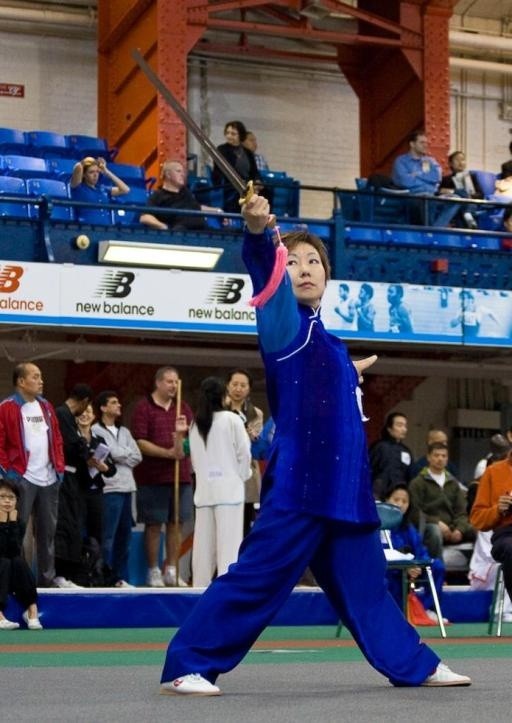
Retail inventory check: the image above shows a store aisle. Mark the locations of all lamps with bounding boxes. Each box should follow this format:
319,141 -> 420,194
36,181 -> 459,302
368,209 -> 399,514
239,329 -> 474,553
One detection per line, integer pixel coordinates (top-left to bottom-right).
97,237 -> 225,271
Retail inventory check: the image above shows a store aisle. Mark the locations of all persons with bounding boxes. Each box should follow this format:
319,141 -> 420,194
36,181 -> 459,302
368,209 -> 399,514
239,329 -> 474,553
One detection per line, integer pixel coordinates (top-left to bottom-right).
212,121 -> 262,213
392,131 -> 462,227
69,156 -> 130,226
439,151 -> 485,229
242,132 -> 268,171
160,194 -> 472,697
139,161 -> 232,229
493,162 -> 512,231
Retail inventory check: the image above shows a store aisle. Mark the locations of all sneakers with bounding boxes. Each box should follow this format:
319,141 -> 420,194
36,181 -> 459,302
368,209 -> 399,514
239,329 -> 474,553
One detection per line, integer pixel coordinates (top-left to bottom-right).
162,565 -> 188,586
114,579 -> 130,588
159,672 -> 224,699
0,616 -> 20,630
145,569 -> 166,588
425,609 -> 449,624
22,609 -> 42,630
38,573 -> 83,588
419,662 -> 472,688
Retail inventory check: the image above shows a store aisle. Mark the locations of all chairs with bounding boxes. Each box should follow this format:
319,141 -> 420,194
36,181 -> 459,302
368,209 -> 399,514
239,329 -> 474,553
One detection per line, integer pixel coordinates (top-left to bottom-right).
338,501 -> 450,639
308,169 -> 510,250
1,129 -> 151,225
188,163 -> 303,240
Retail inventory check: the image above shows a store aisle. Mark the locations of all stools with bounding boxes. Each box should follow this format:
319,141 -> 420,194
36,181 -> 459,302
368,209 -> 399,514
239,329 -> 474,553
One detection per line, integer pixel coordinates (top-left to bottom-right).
486,563 -> 511,639
